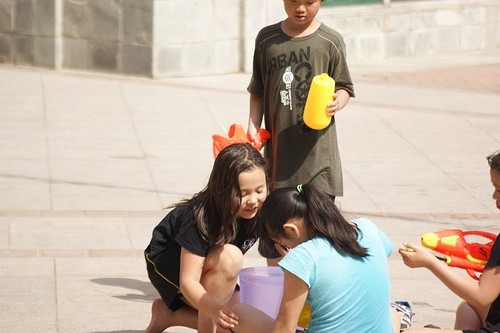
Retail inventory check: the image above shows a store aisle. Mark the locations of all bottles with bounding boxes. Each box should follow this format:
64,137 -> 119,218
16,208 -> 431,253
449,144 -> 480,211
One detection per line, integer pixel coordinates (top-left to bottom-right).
303,73 -> 337,131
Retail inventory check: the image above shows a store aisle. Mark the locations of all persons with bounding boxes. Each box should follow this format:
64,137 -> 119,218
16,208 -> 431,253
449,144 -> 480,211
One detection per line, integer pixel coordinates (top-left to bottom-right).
230,185 -> 394,333
245,1 -> 355,267
397,151 -> 500,333
145,144 -> 268,333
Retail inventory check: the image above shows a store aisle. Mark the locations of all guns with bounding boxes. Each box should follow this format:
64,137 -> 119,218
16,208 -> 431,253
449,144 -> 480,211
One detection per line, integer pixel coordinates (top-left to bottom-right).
212,123 -> 271,161
400,228 -> 497,280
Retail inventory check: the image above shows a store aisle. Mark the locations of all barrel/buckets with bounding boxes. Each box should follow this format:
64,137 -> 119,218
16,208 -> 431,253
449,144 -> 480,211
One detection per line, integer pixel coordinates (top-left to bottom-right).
238,266 -> 285,321
